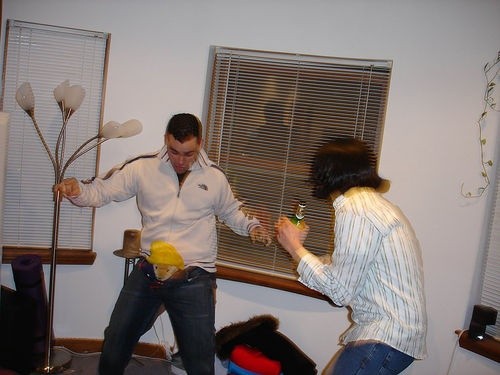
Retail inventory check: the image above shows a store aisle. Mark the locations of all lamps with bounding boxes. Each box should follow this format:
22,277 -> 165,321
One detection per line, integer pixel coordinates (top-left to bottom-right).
16,80 -> 142,375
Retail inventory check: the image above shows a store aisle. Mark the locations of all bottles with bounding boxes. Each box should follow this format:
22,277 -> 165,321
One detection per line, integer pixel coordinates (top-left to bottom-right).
290,200 -> 307,230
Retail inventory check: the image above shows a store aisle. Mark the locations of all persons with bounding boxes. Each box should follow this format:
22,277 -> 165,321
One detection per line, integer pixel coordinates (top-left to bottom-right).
274,135 -> 428,375
52,113 -> 272,375
